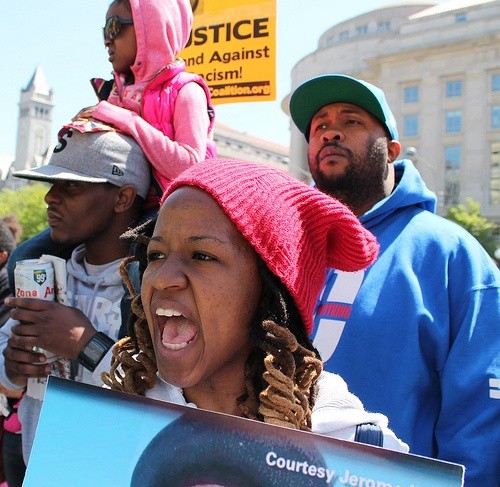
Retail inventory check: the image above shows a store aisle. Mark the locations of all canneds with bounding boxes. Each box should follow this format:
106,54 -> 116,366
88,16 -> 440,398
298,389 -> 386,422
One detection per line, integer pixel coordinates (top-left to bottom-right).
14,258 -> 61,365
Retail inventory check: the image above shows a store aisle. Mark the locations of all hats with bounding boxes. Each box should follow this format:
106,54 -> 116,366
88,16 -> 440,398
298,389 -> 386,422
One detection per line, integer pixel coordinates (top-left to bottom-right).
160,158 -> 380,336
13,131 -> 149,199
289,73 -> 399,143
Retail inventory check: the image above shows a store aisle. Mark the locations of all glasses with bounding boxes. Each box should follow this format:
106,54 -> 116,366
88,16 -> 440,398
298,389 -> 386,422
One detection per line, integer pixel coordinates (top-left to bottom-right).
102,11 -> 133,41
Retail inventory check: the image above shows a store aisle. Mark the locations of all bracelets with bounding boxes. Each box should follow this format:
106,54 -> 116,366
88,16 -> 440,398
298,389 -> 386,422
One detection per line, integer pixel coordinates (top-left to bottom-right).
76,331 -> 116,373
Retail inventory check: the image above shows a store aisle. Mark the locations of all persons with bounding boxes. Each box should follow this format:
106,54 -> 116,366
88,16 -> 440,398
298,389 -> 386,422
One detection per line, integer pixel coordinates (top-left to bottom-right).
290,74 -> 499,487
100,160 -> 409,452
71,0 -> 215,190
0,129 -> 152,487
